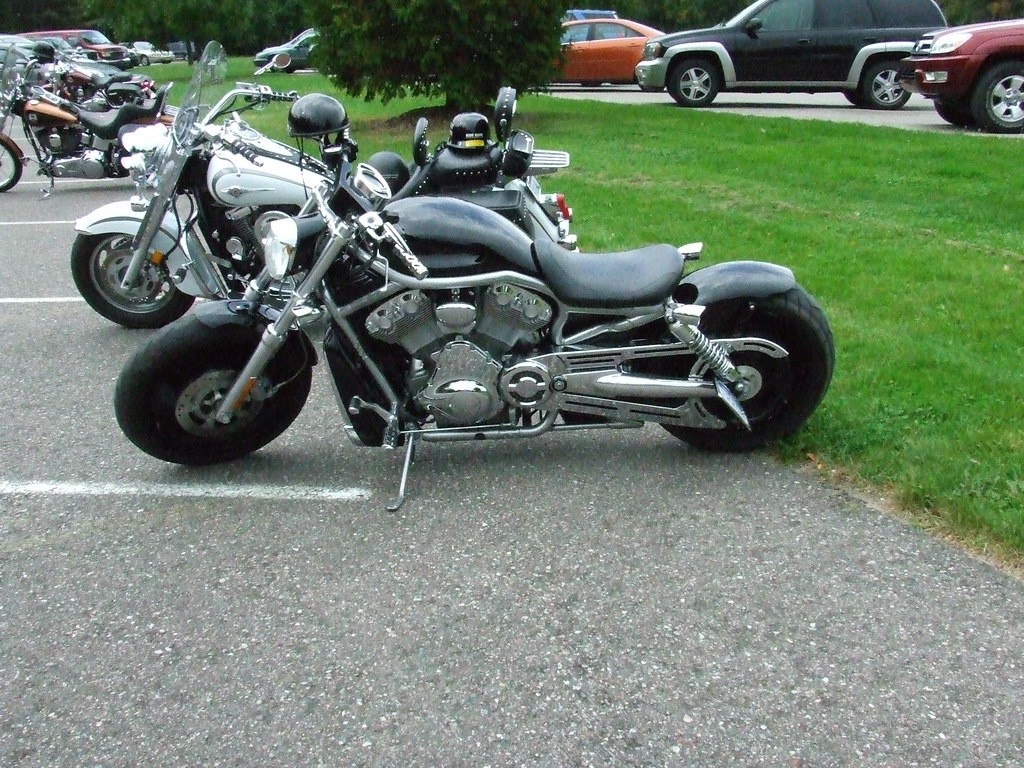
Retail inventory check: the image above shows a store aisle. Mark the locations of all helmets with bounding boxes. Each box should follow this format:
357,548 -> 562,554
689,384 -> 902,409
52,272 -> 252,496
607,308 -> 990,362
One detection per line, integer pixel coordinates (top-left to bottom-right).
288,93 -> 350,136
32,40 -> 55,59
445,112 -> 499,149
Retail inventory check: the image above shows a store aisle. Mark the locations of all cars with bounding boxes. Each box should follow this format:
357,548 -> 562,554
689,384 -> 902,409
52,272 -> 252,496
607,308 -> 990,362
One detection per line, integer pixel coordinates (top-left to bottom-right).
551,19 -> 667,85
0,35 -> 100,79
253,27 -> 319,74
118,42 -> 175,66
166,42 -> 195,61
898,19 -> 1024,135
561,10 -> 629,87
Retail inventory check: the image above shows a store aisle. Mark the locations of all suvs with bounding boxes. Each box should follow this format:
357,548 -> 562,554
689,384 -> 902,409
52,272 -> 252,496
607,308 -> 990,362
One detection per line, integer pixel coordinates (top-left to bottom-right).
634,0 -> 949,110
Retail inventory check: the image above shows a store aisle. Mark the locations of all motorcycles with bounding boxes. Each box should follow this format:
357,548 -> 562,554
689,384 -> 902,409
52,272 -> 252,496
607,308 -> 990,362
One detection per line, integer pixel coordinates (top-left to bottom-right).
114,127 -> 836,511
71,40 -> 580,329
0,40 -> 188,198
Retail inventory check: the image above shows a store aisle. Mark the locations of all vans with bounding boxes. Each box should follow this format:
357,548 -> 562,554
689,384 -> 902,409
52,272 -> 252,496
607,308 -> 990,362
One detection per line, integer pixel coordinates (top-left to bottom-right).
15,30 -> 132,71
